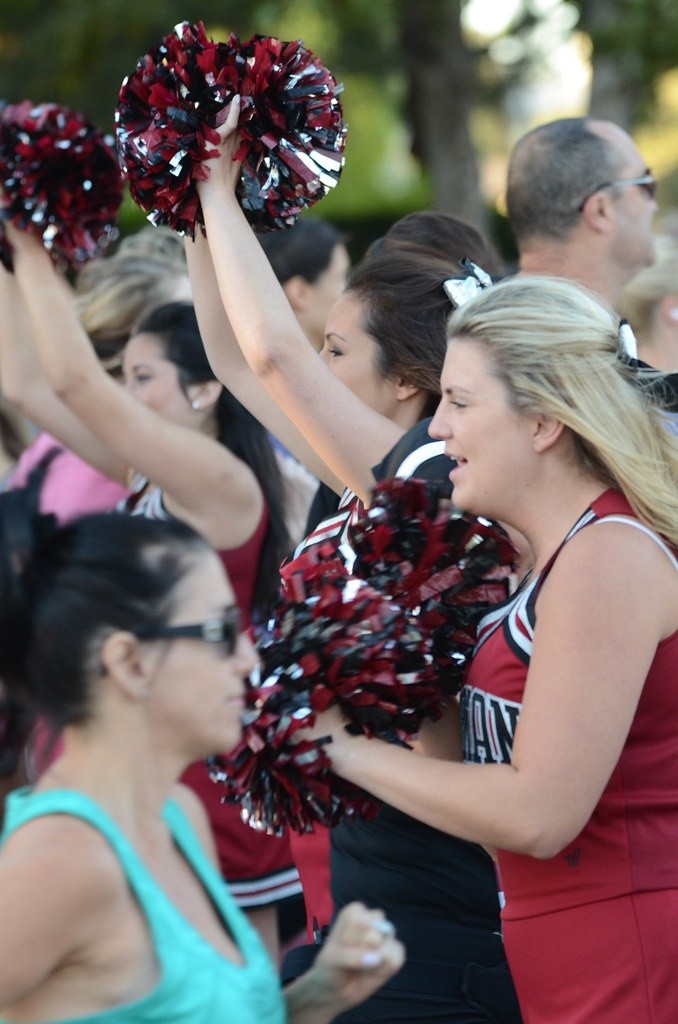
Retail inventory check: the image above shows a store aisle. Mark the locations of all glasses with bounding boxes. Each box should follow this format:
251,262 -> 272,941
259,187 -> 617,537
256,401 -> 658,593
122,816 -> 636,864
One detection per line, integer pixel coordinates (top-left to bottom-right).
579,167 -> 656,212
99,605 -> 243,676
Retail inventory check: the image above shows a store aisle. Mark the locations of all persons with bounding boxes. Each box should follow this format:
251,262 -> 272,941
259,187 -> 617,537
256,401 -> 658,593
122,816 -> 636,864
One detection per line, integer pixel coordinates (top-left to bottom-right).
0,94 -> 678,1024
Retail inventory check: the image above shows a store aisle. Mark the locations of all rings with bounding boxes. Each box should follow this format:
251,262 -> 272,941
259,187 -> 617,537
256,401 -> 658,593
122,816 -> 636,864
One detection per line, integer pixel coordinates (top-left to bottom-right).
371,918 -> 395,939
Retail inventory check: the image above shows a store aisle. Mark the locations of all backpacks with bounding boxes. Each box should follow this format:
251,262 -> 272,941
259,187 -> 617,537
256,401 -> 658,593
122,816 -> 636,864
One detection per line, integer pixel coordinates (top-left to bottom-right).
0,449 -> 74,689
